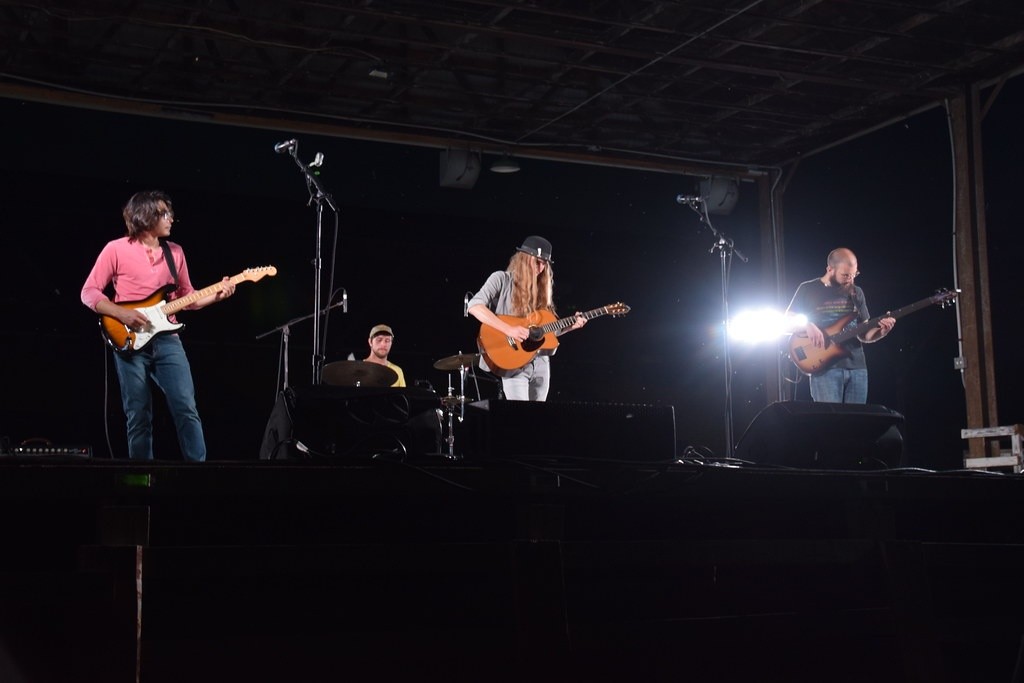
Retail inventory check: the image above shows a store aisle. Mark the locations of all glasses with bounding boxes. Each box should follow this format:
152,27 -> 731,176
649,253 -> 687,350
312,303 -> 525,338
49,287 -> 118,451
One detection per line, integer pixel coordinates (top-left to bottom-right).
159,210 -> 175,220
840,270 -> 860,280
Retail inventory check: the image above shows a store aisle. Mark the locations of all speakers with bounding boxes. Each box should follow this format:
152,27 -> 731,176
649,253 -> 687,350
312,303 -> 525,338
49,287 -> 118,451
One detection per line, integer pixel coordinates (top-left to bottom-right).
486,401 -> 676,465
736,400 -> 905,467
259,386 -> 440,463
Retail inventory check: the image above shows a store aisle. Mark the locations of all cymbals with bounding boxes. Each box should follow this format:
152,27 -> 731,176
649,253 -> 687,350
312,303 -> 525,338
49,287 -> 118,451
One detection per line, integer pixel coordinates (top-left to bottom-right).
321,360 -> 399,386
433,354 -> 479,370
440,396 -> 474,405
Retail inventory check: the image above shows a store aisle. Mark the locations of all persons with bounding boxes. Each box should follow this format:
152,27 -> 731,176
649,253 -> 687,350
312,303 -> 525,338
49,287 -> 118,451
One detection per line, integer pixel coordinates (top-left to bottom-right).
359,324 -> 409,386
467,236 -> 587,402
80,192 -> 236,462
784,248 -> 897,403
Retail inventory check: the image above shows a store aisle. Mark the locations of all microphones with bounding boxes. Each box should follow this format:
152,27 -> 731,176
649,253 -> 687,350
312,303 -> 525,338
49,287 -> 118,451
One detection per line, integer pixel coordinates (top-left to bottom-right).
677,194 -> 702,204
464,294 -> 469,317
274,139 -> 297,153
343,290 -> 347,313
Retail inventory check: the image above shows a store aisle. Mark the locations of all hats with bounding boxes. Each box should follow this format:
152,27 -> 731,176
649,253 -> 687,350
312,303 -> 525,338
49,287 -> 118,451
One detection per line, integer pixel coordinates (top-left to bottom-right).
515,236 -> 554,265
369,324 -> 394,339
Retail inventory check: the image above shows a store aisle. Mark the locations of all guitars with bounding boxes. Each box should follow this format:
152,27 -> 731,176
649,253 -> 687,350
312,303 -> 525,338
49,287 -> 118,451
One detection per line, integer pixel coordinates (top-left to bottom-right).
98,264 -> 278,359
477,302 -> 631,378
788,286 -> 961,376
